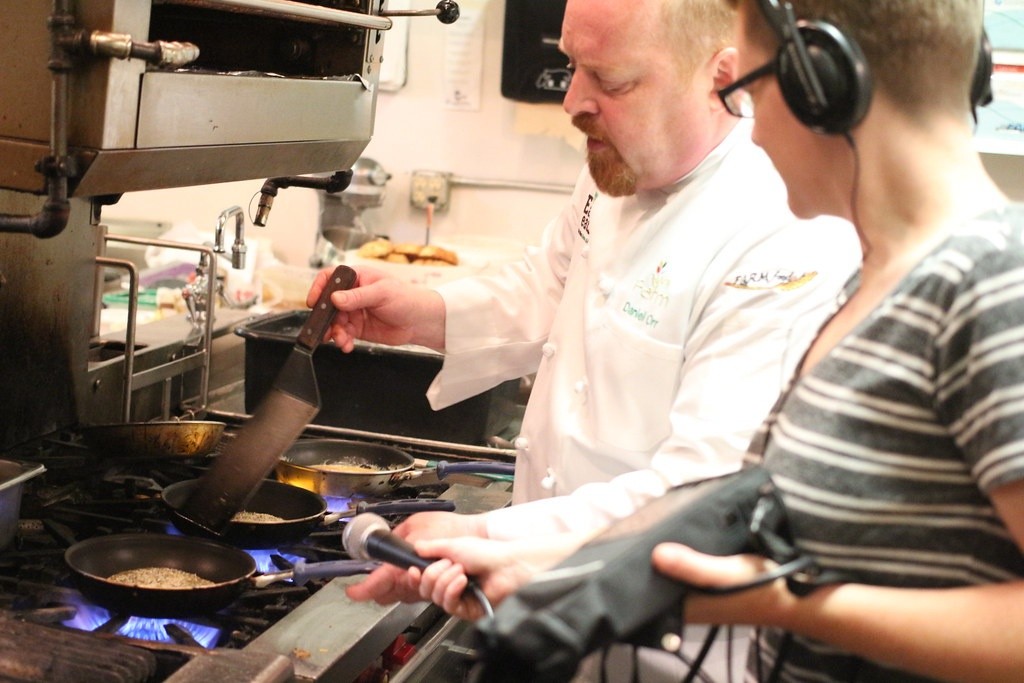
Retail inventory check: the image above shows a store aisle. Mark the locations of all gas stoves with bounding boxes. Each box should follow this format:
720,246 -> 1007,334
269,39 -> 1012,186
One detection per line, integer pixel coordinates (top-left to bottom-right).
0,403 -> 515,683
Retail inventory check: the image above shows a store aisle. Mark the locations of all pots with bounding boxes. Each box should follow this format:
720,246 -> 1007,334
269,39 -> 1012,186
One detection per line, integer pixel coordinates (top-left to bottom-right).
64,532 -> 382,619
84,421 -> 226,465
161,478 -> 455,549
278,438 -> 516,499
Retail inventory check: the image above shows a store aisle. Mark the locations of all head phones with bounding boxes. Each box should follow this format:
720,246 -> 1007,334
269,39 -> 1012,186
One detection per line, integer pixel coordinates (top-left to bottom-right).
760,0 -> 993,135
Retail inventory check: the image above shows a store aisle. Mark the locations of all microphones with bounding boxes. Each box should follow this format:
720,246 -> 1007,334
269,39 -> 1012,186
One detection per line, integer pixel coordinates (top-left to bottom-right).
342,513 -> 480,599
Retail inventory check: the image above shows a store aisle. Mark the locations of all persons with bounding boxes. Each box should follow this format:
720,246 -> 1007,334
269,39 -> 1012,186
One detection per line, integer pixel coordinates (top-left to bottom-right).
307,2 -> 865,683
408,1 -> 1022,683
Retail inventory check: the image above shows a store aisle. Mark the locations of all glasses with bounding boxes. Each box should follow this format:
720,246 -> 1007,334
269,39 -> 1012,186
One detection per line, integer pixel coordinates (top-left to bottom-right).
717,61 -> 775,118
